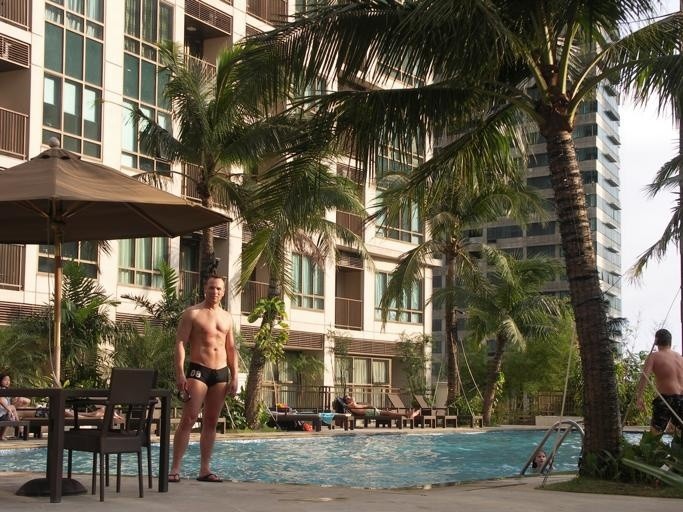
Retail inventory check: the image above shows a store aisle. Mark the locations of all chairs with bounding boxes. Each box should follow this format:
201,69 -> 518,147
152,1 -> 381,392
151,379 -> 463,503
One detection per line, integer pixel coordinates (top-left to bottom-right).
63,365 -> 160,504
121,383 -> 486,436
19,415 -> 125,436
0,417 -> 34,442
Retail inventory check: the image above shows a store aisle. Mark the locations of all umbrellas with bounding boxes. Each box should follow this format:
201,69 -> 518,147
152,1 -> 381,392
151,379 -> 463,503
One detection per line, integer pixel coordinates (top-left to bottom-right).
0,137 -> 236,387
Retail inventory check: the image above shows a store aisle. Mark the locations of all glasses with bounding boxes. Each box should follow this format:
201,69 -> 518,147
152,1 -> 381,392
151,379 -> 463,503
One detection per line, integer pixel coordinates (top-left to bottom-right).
177,389 -> 191,402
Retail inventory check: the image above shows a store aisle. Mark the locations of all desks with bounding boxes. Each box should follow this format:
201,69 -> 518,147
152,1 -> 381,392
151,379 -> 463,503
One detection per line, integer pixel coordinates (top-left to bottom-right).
0,388 -> 174,503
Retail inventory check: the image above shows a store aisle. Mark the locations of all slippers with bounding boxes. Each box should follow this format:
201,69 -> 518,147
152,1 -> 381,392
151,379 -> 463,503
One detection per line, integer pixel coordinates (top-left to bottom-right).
167,473 -> 180,482
197,473 -> 223,482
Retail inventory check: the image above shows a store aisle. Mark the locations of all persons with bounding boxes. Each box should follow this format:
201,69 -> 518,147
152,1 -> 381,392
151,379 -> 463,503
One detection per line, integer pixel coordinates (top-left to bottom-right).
167,273 -> 238,482
10,396 -> 122,420
636,329 -> 681,443
532,449 -> 555,470
344,394 -> 420,419
0,375 -> 23,440
